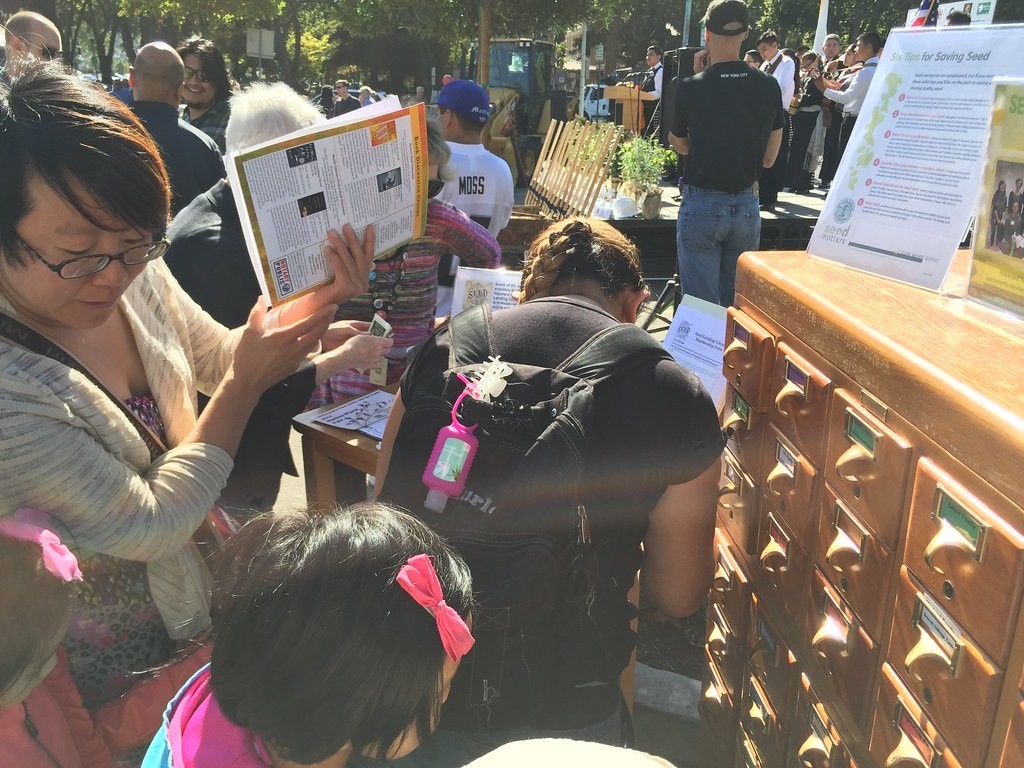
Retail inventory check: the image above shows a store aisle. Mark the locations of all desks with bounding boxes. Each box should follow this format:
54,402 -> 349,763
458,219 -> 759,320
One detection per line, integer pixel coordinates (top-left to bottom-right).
293,382 -> 402,516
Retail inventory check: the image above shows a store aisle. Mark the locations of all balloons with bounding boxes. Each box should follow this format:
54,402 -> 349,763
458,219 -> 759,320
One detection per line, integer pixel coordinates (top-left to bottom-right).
442,74 -> 453,85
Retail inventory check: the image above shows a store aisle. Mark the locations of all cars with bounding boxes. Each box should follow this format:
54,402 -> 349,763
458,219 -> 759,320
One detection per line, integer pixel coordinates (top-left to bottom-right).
583,83 -> 610,124
609,67 -> 653,91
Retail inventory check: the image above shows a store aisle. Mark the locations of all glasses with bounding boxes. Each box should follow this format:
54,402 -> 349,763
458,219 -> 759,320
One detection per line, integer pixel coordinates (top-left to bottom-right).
337,86 -> 343,89
183,66 -> 213,82
800,59 -> 814,72
428,175 -> 444,199
746,59 -> 756,63
18,39 -> 67,61
13,231 -> 170,282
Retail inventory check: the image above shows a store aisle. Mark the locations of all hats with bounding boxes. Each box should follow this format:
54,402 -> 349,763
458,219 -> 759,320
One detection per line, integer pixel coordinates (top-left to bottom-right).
441,75 -> 453,84
437,79 -> 490,123
699,0 -> 749,36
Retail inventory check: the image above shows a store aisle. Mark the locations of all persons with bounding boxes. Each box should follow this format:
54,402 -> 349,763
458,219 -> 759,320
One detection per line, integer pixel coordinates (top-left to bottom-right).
343,217 -> 726,768
669,0 -> 783,306
991,177 -> 1024,246
0,507 -> 216,768
408,87 -> 426,106
163,81 -> 390,516
0,62 -> 375,768
431,80 -> 513,317
0,11 -> 231,224
294,119 -> 502,509
134,500 -> 473,768
315,79 -> 376,119
640,12 -> 971,209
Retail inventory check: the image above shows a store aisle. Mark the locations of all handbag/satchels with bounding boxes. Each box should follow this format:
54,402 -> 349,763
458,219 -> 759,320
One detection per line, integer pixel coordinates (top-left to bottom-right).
51,503 -> 237,765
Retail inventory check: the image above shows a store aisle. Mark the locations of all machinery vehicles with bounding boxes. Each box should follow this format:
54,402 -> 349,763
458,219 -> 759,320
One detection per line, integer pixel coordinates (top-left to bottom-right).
467,35 -> 580,190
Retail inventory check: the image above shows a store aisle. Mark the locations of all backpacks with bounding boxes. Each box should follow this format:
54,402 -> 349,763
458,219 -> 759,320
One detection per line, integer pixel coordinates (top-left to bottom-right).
415,303 -> 675,732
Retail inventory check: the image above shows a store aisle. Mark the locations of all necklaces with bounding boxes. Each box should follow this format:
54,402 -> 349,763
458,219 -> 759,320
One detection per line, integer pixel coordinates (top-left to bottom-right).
368,246 -> 409,320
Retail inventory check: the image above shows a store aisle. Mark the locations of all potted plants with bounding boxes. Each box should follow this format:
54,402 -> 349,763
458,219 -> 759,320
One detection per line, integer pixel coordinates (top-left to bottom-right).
616,133 -> 677,221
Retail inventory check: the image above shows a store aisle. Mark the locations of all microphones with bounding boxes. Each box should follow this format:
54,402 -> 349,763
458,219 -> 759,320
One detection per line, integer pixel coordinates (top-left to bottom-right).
629,71 -> 655,77
615,67 -> 632,72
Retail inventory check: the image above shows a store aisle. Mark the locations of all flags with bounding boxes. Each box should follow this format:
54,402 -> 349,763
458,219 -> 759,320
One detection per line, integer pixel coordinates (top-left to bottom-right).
911,0 -> 939,27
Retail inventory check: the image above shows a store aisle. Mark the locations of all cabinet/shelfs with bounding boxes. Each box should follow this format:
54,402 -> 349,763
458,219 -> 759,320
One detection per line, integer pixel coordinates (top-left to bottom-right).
701,245 -> 1024,768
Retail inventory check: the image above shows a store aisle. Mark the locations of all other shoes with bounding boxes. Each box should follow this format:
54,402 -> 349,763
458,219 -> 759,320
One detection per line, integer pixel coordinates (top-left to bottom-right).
810,184 -> 814,190
818,181 -> 831,189
670,178 -> 678,185
674,195 -> 683,201
795,188 -> 809,195
662,174 -> 674,182
760,200 -> 777,211
